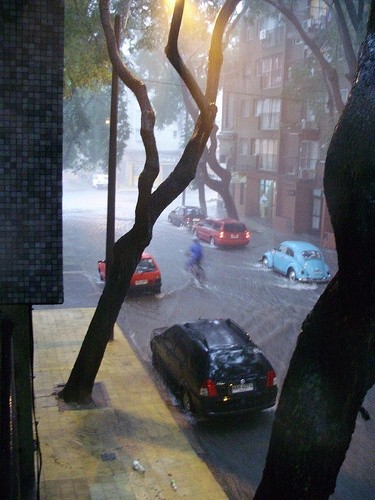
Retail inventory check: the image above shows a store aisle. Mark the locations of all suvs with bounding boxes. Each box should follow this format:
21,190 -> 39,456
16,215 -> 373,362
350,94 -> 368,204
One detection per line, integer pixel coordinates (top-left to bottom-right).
191,216 -> 251,249
149,316 -> 279,423
97,250 -> 161,296
167,205 -> 207,229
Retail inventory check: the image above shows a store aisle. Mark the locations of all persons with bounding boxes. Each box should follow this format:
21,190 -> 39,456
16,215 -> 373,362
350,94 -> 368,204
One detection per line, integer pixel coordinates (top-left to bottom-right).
183,237 -> 203,274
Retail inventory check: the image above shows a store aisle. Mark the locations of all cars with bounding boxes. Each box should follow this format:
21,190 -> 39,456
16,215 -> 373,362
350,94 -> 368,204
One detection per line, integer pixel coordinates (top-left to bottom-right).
92,174 -> 108,190
260,240 -> 331,284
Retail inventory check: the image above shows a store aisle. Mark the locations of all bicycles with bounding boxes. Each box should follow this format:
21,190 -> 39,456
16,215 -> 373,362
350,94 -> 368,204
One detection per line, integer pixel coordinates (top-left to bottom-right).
184,250 -> 207,283
